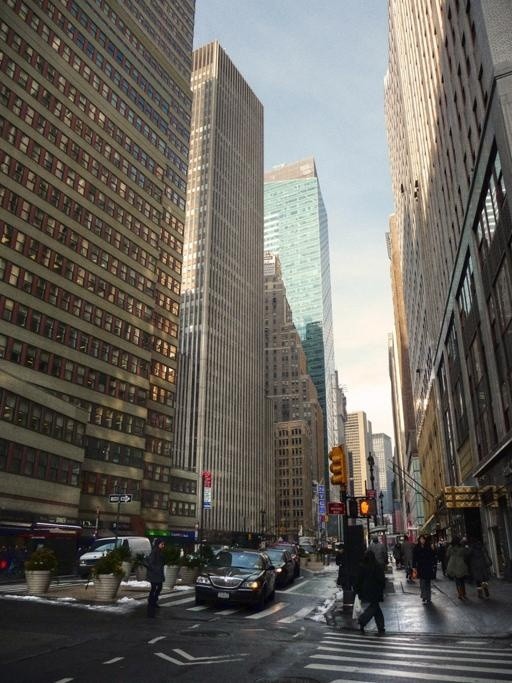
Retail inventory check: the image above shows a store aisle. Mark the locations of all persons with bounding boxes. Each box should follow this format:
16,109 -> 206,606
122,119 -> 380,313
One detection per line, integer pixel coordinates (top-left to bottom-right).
336,544 -> 346,585
356,550 -> 388,635
367,535 -> 389,600
393,533 -> 492,606
144,537 -> 168,619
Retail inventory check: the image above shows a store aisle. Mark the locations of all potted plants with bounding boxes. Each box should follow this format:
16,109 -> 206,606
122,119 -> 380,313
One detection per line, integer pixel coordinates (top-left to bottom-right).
23,538 -> 215,604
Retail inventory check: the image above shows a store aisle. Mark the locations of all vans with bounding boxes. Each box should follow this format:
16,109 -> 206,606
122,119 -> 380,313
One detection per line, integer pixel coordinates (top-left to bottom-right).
79,536 -> 153,573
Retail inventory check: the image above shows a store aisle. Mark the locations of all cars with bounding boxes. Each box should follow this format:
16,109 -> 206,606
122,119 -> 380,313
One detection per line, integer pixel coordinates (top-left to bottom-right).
195,542 -> 301,611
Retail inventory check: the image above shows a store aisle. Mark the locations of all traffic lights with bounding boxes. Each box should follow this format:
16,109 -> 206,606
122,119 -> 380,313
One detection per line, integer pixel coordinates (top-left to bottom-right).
349,495 -> 373,520
330,446 -> 348,488
